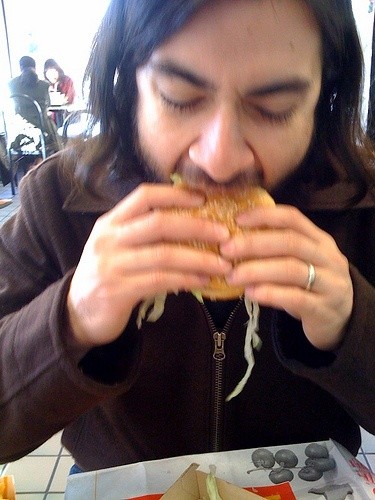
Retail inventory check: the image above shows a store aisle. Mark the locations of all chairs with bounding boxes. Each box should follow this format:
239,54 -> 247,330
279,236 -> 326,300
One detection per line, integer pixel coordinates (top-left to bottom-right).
2,93 -> 65,195
62,109 -> 101,147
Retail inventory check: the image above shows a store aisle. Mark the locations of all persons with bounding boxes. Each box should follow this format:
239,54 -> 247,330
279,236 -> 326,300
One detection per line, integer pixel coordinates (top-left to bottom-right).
0,0 -> 375,476
45,59 -> 75,127
9,56 -> 57,152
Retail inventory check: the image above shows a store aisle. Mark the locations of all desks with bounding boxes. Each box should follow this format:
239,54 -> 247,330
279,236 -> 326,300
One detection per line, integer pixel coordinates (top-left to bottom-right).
47,105 -> 68,128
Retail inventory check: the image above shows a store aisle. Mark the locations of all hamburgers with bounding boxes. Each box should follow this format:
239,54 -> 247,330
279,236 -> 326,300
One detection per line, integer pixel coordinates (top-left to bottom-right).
150,170 -> 278,302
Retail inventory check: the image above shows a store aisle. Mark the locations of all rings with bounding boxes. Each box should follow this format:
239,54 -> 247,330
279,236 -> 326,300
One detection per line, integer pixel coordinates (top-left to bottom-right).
307,261 -> 315,290
